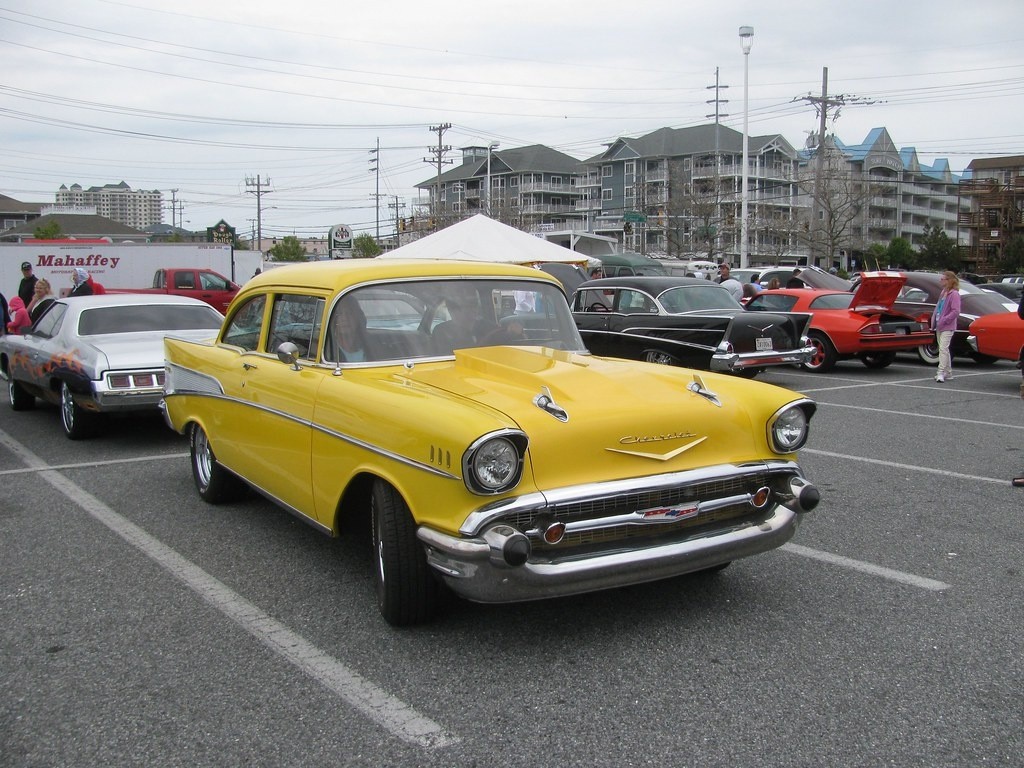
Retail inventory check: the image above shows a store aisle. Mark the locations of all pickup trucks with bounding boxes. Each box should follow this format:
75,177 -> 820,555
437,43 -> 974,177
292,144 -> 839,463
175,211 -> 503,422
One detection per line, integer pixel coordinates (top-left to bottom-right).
58,267 -> 260,328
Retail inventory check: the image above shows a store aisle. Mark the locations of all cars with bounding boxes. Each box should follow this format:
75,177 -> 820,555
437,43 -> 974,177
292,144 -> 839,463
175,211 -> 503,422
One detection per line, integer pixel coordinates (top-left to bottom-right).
494,245 -> 1024,381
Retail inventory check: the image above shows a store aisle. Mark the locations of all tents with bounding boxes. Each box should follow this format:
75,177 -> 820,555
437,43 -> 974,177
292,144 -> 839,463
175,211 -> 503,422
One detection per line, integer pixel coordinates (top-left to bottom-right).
377,212 -> 606,277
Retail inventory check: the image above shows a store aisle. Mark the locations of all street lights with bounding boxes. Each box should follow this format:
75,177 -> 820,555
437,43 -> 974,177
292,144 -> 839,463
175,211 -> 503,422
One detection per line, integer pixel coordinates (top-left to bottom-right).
257,205 -> 278,252
735,26 -> 756,269
487,141 -> 502,216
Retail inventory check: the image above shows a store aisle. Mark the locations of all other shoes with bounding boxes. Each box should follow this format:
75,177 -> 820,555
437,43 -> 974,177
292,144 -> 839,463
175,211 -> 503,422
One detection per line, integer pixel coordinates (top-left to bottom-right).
0,369 -> 8,382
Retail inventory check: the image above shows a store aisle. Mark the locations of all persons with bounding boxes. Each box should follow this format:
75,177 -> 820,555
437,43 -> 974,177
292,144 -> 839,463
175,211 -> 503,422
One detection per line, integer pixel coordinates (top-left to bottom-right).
332,294 -> 391,363
67,268 -> 105,296
431,286 -> 528,355
750,274 -> 762,290
590,268 -> 602,280
713,262 -> 757,302
7,297 -> 33,336
829,267 -> 838,275
931,270 -> 960,383
26,278 -> 56,325
768,275 -> 780,290
1017,284 -> 1024,400
514,290 -> 536,312
786,269 -> 804,288
19,261 -> 39,308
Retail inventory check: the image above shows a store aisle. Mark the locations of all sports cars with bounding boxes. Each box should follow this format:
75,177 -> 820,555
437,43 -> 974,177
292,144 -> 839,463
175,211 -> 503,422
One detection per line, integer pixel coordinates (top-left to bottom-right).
0,293 -> 252,439
155,256 -> 822,627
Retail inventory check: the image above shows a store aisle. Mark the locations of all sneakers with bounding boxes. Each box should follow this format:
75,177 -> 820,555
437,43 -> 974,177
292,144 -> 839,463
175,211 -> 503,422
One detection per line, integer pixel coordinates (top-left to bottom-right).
935,373 -> 953,380
937,375 -> 944,383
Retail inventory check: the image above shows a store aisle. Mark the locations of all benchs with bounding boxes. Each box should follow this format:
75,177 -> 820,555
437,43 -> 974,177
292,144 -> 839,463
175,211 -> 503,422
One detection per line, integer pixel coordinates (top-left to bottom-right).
268,324 -> 436,362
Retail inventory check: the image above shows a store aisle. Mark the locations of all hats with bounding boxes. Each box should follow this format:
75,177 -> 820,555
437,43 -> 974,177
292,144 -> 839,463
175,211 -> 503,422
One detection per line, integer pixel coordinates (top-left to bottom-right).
718,263 -> 730,269
21,262 -> 32,270
